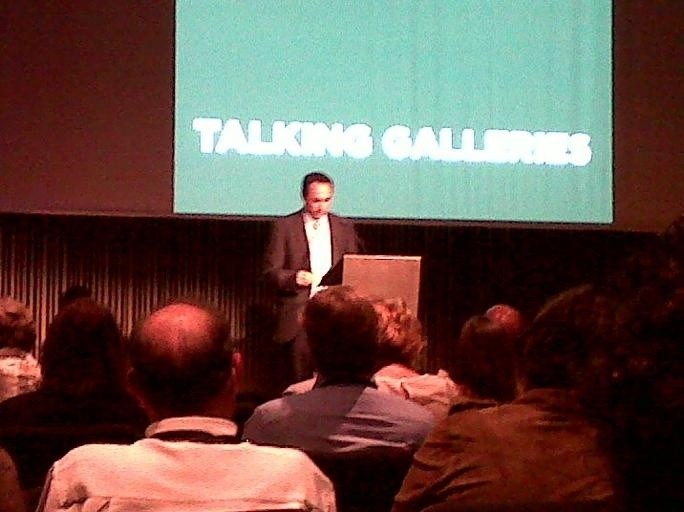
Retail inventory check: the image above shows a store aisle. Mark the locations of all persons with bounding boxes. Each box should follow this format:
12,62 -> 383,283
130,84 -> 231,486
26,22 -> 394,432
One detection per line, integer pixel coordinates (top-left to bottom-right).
1,219 -> 683,511
260,174 -> 359,387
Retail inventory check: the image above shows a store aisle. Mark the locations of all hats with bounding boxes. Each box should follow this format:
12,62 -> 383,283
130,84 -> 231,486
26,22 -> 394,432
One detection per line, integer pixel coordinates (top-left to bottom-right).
0,297 -> 34,328
